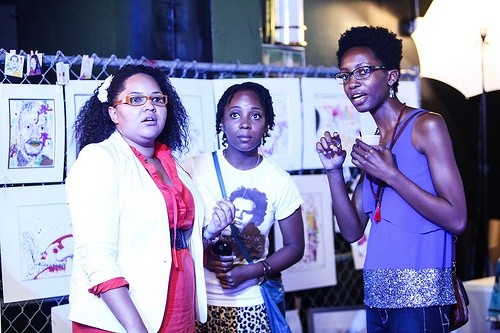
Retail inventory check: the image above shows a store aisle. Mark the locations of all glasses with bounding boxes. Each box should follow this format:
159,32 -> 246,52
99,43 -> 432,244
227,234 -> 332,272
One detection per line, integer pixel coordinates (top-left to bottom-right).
113,95 -> 168,107
334,65 -> 393,84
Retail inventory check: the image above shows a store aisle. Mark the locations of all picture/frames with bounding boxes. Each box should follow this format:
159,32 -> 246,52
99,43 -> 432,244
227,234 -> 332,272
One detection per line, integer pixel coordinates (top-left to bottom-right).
308,306 -> 366,333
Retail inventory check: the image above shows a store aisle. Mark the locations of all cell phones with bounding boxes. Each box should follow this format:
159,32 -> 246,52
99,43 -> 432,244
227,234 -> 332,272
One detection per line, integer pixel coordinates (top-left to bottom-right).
212,241 -> 232,256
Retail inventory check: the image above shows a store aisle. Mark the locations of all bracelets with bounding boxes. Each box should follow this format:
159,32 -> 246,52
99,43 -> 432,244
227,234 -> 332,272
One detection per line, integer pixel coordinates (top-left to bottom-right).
203,224 -> 215,245
260,260 -> 271,275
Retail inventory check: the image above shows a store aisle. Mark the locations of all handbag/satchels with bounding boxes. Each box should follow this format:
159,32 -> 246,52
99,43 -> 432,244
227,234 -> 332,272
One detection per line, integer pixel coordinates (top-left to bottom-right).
259,277 -> 292,333
449,273 -> 469,331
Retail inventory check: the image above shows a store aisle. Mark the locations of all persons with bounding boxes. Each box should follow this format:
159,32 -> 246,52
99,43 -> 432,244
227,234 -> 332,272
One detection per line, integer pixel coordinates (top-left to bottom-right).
219,187 -> 268,238
65,63 -> 234,333
182,82 -> 306,333
316,25 -> 468,333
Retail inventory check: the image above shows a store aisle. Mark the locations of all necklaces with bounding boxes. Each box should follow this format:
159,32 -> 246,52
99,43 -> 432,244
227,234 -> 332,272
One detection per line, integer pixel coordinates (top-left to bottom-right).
368,102 -> 407,221
224,148 -> 261,203
